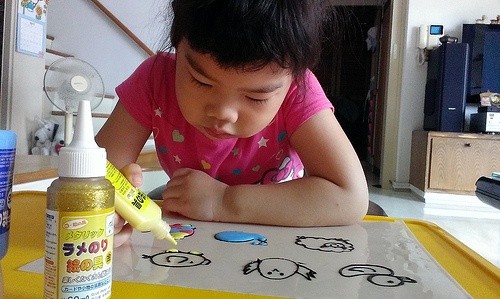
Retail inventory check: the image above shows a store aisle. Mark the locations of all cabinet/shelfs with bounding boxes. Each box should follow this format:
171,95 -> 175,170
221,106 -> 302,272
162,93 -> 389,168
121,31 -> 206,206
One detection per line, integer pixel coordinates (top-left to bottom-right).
409,129 -> 500,203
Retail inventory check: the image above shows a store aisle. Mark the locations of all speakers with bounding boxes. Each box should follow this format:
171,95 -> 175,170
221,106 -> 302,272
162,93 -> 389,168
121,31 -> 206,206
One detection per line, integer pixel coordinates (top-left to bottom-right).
423,43 -> 469,132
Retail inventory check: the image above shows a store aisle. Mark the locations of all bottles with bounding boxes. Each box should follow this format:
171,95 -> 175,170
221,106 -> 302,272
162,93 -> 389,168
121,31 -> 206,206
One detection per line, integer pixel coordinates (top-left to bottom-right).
43,99 -> 116,299
104,159 -> 177,247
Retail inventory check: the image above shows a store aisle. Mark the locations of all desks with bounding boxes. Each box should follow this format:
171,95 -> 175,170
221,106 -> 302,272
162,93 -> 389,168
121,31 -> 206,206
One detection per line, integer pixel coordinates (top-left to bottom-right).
0,190 -> 500,299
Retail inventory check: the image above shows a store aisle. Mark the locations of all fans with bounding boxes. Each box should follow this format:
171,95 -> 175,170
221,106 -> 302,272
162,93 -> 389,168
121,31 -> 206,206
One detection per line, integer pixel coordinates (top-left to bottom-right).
43,57 -> 105,146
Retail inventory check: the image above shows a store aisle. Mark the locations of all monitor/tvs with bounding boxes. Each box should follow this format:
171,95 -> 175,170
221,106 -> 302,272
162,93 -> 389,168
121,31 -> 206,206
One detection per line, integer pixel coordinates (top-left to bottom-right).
463,24 -> 500,103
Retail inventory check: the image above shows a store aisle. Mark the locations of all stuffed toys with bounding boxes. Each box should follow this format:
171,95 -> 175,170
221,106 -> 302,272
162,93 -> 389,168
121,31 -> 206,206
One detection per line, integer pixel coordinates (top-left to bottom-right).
30,122 -> 56,156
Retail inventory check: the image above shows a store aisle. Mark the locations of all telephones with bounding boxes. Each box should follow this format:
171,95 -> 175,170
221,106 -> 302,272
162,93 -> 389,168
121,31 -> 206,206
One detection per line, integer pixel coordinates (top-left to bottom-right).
417,23 -> 444,65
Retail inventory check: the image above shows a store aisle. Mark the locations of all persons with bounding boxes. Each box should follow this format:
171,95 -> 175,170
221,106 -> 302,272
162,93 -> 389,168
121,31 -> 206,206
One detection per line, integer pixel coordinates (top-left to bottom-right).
95,0 -> 368,249
